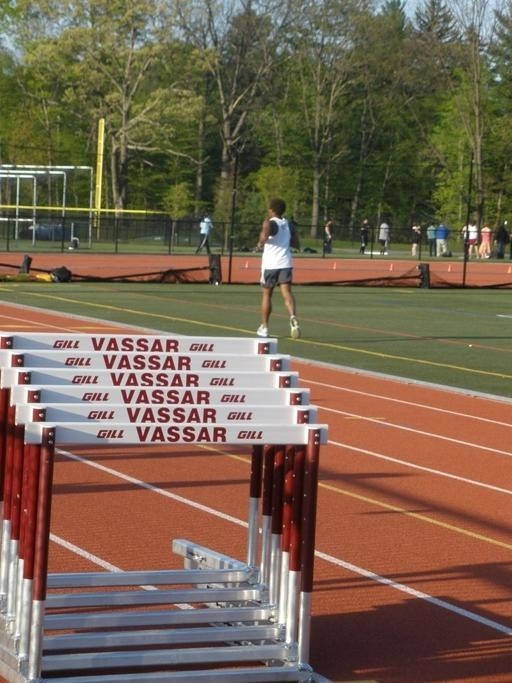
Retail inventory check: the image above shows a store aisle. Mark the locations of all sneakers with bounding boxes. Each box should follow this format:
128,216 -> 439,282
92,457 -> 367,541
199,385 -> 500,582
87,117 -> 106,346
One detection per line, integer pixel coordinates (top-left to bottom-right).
289,318 -> 301,338
255,325 -> 270,338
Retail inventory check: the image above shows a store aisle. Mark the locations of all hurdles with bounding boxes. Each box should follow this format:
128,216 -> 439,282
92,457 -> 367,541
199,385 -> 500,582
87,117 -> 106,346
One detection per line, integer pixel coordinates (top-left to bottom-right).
1,384 -> 312,647
0,330 -> 281,587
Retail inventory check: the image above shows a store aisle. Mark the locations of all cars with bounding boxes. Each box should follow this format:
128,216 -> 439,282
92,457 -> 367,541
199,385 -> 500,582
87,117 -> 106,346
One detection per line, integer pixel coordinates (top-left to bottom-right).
18,212 -> 82,242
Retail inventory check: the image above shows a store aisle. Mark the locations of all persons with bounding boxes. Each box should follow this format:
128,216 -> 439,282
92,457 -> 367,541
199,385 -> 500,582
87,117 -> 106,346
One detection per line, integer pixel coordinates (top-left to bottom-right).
378,218 -> 390,255
322,218 -> 333,254
410,219 -> 451,258
461,219 -> 512,259
256,198 -> 302,339
358,217 -> 368,255
193,212 -> 214,255
